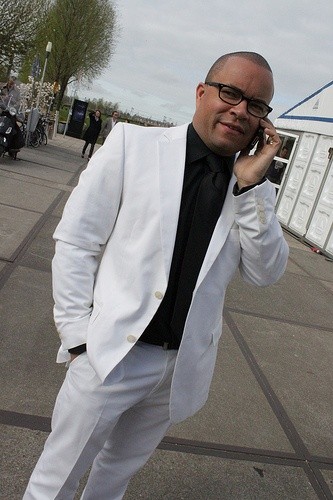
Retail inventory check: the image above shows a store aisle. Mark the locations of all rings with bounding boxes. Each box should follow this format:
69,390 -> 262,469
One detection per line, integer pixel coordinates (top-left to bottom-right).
269,141 -> 274,144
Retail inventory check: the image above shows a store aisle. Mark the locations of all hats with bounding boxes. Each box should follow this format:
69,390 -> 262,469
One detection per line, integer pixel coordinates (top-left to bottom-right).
9,73 -> 17,78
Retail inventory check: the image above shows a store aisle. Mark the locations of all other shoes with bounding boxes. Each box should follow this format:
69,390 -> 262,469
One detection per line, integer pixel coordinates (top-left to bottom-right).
80,154 -> 84,159
87,158 -> 90,161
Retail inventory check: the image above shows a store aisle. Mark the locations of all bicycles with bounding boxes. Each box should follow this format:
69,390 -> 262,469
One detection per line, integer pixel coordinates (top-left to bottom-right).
29,117 -> 47,148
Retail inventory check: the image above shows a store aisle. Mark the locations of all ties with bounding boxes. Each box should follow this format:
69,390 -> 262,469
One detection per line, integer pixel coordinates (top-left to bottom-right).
174,154 -> 228,310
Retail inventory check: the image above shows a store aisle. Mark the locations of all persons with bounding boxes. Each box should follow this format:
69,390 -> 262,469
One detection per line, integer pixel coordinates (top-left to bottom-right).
22,51 -> 290,500
82,109 -> 130,161
268,147 -> 288,196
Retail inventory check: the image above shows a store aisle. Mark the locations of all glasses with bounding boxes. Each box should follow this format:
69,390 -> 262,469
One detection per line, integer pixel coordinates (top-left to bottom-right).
205,80 -> 273,118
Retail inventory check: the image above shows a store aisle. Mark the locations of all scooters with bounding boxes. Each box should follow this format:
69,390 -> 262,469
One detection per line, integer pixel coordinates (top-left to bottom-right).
0,91 -> 26,160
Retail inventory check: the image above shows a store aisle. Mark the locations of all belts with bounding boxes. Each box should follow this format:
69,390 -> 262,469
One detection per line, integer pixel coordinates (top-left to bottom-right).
139,331 -> 181,351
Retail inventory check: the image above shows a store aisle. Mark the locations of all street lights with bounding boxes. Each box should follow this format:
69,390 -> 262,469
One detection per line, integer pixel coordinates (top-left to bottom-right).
24,41 -> 52,147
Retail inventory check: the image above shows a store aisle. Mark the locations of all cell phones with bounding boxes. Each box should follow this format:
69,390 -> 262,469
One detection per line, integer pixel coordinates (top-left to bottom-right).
248,126 -> 264,149
